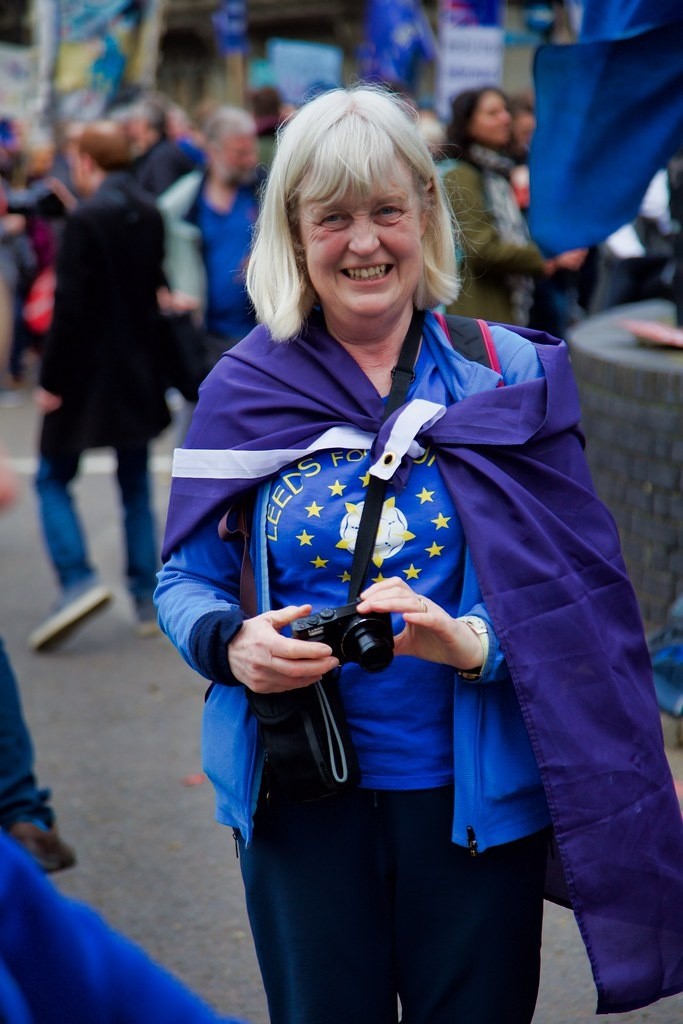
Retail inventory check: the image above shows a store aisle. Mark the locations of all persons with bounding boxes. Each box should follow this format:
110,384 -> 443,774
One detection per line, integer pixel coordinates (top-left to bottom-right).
0,0 -> 683,1024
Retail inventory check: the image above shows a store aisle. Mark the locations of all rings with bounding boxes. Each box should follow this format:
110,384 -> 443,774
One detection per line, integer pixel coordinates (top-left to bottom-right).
417,598 -> 428,613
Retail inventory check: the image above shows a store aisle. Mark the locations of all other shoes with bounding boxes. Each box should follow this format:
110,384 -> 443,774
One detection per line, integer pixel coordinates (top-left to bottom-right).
30,583 -> 114,652
137,608 -> 161,637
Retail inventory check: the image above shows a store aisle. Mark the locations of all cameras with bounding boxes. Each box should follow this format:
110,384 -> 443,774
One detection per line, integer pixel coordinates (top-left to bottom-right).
291,599 -> 394,672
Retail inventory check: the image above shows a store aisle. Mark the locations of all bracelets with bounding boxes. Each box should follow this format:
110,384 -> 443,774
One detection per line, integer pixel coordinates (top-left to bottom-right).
452,615 -> 488,680
